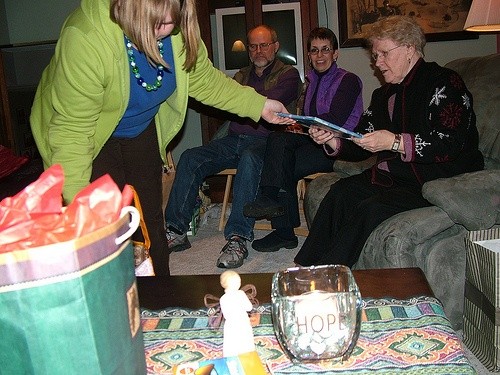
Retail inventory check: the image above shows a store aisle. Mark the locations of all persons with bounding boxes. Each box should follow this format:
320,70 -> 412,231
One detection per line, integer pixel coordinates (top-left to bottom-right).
29,0 -> 296,276
218,271 -> 255,358
164,24 -> 301,269
245,27 -> 365,252
293,15 -> 484,270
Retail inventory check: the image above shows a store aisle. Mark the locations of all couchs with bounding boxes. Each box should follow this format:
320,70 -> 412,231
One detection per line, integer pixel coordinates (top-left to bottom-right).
303,53 -> 500,331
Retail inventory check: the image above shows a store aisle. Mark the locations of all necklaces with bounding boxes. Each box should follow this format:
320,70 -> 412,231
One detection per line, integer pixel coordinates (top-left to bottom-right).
123,33 -> 164,91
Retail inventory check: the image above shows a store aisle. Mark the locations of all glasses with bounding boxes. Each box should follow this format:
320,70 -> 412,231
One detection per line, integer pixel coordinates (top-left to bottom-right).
248,40 -> 278,52
308,47 -> 333,55
372,44 -> 406,61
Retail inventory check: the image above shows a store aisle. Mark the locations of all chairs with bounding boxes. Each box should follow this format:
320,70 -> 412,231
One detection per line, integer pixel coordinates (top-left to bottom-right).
215,169 -> 328,237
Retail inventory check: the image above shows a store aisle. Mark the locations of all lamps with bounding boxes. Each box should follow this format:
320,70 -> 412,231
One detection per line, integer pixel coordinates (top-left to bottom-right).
463,0 -> 500,32
232,39 -> 246,52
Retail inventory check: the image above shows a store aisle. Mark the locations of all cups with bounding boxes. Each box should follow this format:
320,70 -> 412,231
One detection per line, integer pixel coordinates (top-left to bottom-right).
270,265 -> 362,370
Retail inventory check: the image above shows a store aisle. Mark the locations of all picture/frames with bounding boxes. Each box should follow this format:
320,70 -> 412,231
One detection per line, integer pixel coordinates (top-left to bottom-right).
337,0 -> 479,48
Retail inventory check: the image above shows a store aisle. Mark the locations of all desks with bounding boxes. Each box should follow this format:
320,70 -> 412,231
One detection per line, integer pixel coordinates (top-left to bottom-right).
137,267 -> 479,375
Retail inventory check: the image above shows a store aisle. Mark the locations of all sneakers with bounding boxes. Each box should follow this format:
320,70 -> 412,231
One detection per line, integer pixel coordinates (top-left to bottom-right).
165,229 -> 191,254
253,230 -> 298,253
242,197 -> 285,219
217,238 -> 248,269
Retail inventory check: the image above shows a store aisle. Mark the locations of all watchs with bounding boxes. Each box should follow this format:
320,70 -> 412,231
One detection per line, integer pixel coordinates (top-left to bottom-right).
391,133 -> 401,152
